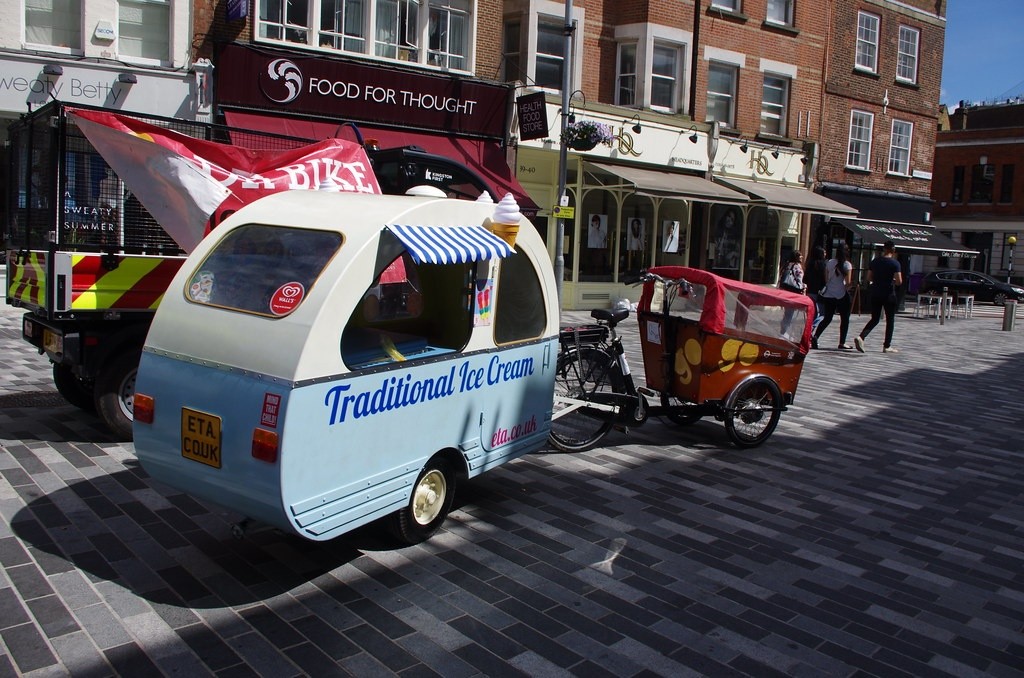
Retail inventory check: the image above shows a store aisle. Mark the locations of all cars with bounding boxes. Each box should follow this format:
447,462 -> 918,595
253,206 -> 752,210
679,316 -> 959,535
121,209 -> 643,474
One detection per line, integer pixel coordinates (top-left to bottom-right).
917,270 -> 1024,306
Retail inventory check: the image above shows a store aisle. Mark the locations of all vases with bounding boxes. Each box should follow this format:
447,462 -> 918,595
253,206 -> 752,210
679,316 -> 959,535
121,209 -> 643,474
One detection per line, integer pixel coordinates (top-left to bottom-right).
572,140 -> 597,151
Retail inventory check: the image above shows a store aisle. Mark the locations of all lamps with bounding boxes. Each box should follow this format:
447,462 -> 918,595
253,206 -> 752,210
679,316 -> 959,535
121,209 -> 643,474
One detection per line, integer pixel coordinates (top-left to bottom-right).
624,114 -> 641,134
119,73 -> 137,83
764,143 -> 779,159
559,103 -> 575,123
732,136 -> 747,153
793,149 -> 810,165
43,64 -> 63,75
681,125 -> 697,143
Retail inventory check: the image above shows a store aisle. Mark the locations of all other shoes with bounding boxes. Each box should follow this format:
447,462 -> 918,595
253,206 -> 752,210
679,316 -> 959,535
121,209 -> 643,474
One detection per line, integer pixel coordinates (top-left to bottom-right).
838,345 -> 853,349
812,337 -> 818,349
854,336 -> 865,353
883,346 -> 898,353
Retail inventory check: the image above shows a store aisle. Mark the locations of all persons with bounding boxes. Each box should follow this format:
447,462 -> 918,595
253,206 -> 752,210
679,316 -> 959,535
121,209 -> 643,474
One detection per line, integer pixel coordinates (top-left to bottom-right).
812,242 -> 852,350
13,213 -> 19,238
779,250 -> 808,341
664,222 -> 675,251
589,214 -> 606,248
854,240 -> 902,353
802,246 -> 827,349
629,218 -> 643,250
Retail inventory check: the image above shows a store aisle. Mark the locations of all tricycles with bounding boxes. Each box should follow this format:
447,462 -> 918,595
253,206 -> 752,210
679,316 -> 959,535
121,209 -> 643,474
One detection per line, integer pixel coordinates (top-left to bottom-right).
547,267 -> 815,454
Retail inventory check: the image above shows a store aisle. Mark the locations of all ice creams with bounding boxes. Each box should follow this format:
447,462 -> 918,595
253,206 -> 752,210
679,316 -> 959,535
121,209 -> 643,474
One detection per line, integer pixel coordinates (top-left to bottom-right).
476,190 -> 493,202
493,192 -> 520,248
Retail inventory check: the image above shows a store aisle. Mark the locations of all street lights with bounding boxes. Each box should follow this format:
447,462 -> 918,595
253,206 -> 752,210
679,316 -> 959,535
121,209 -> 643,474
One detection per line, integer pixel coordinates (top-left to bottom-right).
1007,237 -> 1017,285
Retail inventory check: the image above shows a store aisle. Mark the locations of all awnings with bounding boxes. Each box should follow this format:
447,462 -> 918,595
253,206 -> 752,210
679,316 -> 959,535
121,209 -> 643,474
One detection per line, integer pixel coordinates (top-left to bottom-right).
712,173 -> 859,220
837,217 -> 980,260
225,110 -> 543,221
577,153 -> 751,209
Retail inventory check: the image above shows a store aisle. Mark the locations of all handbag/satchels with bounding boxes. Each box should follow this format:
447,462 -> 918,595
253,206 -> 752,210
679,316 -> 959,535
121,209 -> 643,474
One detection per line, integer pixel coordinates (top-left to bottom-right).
780,264 -> 802,291
817,291 -> 823,302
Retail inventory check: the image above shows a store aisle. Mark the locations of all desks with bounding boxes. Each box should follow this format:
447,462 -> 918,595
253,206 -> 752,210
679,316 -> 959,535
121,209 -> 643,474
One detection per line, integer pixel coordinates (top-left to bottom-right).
958,296 -> 974,319
917,294 -> 952,320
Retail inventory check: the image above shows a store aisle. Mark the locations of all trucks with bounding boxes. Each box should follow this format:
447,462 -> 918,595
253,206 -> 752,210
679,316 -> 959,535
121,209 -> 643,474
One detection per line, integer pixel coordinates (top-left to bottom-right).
23,121 -> 561,545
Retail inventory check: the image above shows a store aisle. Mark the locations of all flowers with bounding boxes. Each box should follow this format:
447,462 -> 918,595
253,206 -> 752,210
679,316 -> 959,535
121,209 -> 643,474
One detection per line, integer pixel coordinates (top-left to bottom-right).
560,121 -> 615,151
